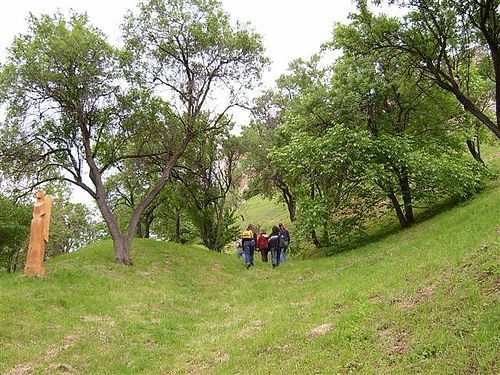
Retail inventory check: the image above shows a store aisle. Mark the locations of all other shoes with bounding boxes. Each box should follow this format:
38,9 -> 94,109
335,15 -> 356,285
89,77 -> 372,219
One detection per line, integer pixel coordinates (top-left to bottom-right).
272,264 -> 276,267
247,264 -> 250,269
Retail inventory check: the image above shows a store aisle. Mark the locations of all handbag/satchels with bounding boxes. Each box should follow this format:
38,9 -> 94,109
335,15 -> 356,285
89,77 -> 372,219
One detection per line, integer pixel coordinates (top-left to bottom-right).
278,232 -> 285,247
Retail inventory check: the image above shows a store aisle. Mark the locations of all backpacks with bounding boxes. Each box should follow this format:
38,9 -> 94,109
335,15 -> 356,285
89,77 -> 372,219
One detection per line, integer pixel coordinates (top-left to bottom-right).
243,230 -> 254,241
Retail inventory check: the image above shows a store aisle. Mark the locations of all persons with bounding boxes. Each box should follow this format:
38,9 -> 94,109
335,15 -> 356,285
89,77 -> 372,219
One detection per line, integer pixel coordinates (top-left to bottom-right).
235,234 -> 247,264
241,224 -> 257,268
257,228 -> 270,262
278,223 -> 290,263
267,225 -> 282,268
